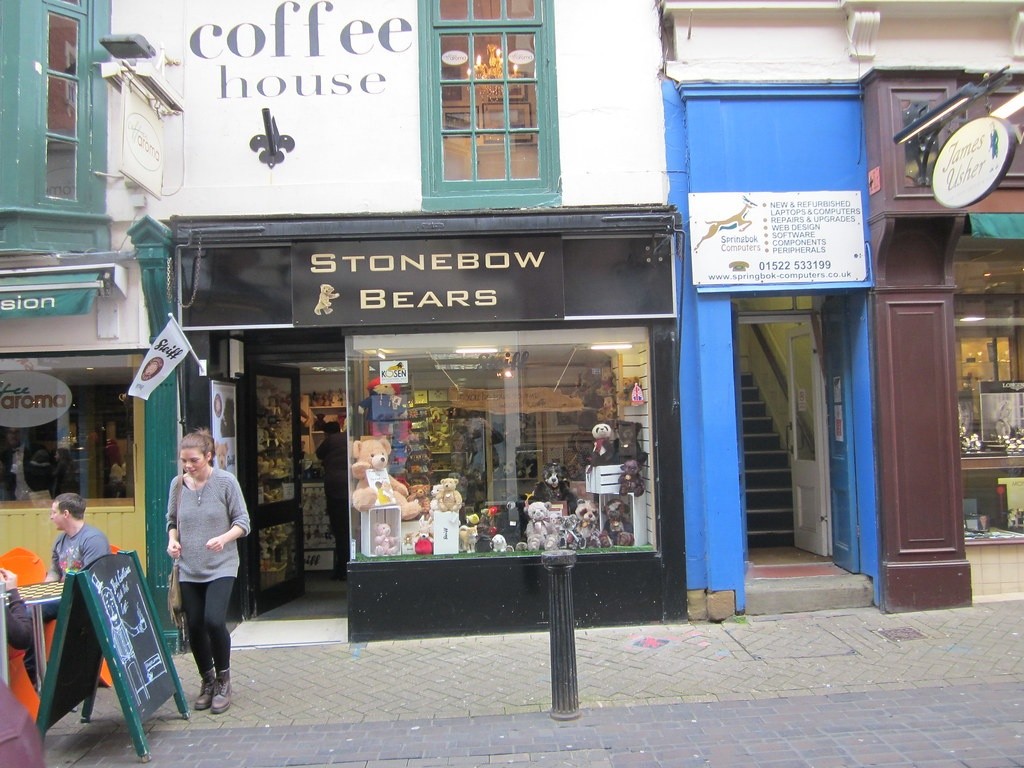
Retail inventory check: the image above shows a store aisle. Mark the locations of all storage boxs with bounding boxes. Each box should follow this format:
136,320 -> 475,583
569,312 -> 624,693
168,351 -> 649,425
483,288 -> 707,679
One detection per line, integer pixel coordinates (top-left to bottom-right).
598,490 -> 648,546
359,395 -> 411,472
360,505 -> 400,557
586,464 -> 641,495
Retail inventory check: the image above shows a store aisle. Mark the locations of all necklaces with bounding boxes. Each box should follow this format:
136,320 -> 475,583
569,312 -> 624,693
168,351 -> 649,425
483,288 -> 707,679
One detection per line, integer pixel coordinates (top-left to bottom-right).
194,467 -> 211,506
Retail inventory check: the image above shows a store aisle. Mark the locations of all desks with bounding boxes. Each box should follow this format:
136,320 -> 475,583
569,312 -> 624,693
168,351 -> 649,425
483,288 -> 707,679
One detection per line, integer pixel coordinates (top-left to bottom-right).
3,580 -> 64,692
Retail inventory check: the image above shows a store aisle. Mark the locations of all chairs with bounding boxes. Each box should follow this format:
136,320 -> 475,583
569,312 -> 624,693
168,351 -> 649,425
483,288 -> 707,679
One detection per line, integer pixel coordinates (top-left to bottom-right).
0,544 -> 121,724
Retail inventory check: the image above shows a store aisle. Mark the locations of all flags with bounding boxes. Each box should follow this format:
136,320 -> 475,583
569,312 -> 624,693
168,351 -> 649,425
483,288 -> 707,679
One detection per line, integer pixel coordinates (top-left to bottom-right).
126,318 -> 193,400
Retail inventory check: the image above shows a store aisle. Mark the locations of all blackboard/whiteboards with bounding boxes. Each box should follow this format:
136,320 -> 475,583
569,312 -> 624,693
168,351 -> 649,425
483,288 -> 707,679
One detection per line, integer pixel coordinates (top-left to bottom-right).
78,551 -> 179,725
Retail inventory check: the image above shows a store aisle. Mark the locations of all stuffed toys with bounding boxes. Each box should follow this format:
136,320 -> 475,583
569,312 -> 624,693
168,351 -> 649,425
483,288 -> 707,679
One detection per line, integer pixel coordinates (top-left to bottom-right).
353,365 -> 648,556
257,374 -> 352,503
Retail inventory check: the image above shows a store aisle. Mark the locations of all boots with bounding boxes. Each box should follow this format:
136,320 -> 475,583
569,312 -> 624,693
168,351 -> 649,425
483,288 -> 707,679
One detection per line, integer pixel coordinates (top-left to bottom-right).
211,668 -> 232,714
195,666 -> 215,710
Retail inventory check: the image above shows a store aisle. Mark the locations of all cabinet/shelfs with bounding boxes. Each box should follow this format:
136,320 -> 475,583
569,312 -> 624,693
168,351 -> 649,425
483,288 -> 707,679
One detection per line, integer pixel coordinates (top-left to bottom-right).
309,406 -> 347,453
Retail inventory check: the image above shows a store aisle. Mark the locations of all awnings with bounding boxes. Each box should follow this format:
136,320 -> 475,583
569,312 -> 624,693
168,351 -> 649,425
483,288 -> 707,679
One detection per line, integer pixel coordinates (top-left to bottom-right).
1,264 -> 128,316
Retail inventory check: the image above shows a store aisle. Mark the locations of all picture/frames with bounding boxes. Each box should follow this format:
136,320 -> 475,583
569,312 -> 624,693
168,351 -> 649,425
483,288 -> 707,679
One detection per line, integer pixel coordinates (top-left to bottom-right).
442,105 -> 480,138
442,66 -> 462,101
482,102 -> 533,142
488,71 -> 528,102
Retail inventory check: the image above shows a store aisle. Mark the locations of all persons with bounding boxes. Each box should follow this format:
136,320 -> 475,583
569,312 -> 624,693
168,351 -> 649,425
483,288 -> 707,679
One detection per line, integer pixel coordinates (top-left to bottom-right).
314,421 -> 349,582
165,426 -> 251,714
0,567 -> 32,666
979,515 -> 988,531
51,448 -> 80,499
995,400 -> 1016,440
0,428 -> 61,500
460,412 -> 504,511
23,494 -> 111,691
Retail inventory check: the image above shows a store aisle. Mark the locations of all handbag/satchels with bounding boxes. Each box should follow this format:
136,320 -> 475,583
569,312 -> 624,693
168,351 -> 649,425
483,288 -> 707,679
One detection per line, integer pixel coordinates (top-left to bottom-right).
168,564 -> 185,628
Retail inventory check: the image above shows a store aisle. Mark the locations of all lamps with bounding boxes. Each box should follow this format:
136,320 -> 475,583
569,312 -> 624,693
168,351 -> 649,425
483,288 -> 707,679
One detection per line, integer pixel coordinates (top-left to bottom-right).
892,81 -> 978,144
467,0 -> 519,103
98,32 -> 155,59
